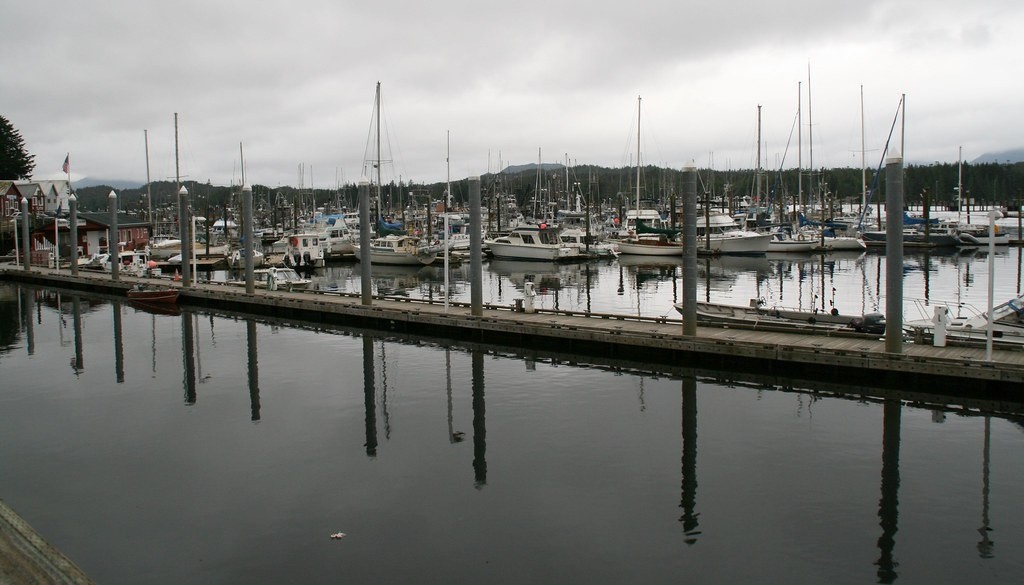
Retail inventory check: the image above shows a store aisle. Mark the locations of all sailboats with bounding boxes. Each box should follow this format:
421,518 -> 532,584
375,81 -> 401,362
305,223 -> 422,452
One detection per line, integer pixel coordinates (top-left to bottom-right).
23,58 -> 1024,344
